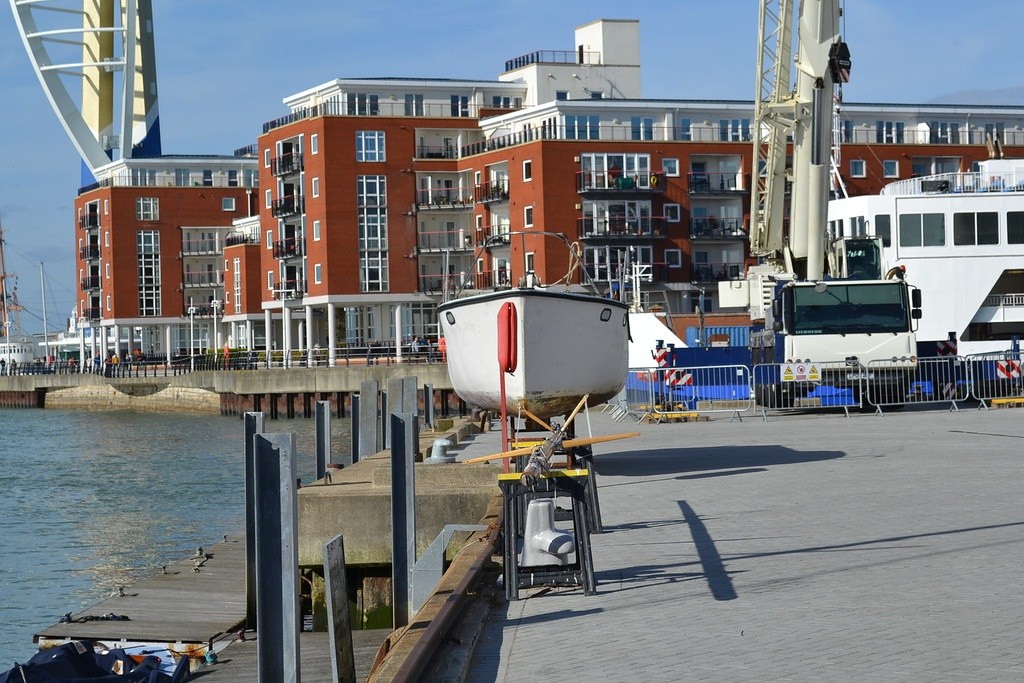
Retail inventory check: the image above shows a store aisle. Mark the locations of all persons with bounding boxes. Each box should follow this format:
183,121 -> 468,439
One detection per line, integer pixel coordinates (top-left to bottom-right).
368,336 -> 433,365
0,353 -> 147,373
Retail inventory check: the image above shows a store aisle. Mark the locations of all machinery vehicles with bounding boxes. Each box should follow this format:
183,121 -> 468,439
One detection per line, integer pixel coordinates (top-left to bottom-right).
745,1 -> 925,415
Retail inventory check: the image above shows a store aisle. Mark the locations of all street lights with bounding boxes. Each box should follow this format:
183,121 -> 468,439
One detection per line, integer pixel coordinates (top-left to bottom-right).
188,297 -> 196,370
270,279 -> 295,371
209,288 -> 222,364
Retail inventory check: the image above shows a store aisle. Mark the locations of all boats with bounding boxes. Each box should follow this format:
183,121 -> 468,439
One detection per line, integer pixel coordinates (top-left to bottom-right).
434,230 -> 632,414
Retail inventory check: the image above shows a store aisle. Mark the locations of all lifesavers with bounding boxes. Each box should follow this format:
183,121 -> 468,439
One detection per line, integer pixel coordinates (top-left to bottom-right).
223,345 -> 230,367
943,382 -> 955,400
936,337 -> 957,359
996,359 -> 1021,379
